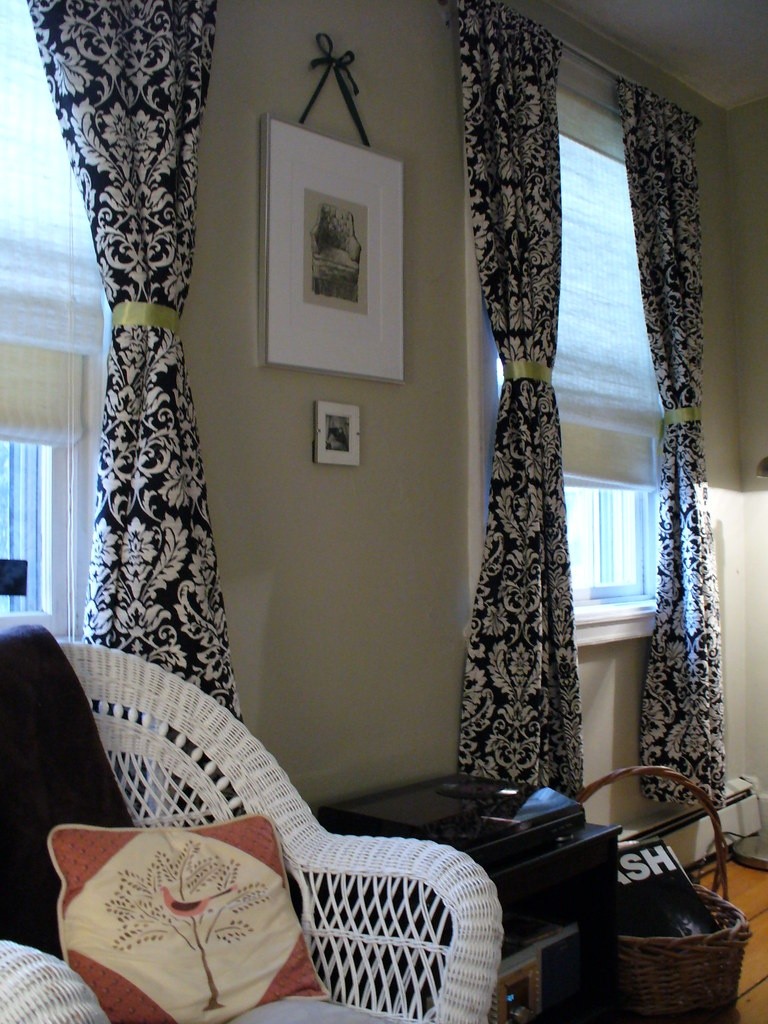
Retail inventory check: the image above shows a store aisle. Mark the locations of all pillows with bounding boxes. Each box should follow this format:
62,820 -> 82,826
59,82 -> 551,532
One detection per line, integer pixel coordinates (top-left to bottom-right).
50,820 -> 329,1024
0,625 -> 138,958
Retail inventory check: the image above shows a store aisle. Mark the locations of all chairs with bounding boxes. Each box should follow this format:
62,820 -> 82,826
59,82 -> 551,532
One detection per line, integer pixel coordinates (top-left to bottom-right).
0,644 -> 504,1024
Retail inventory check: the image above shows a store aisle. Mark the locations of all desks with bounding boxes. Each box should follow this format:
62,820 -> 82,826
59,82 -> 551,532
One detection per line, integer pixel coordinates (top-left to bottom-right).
475,824 -> 625,1024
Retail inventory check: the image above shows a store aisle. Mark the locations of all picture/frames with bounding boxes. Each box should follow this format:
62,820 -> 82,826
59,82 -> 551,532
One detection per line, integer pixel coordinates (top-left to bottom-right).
254,116 -> 405,384
313,402 -> 360,466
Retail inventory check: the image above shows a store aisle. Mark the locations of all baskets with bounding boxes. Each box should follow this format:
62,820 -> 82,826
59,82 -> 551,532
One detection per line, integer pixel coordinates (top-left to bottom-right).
574,765 -> 751,1015
